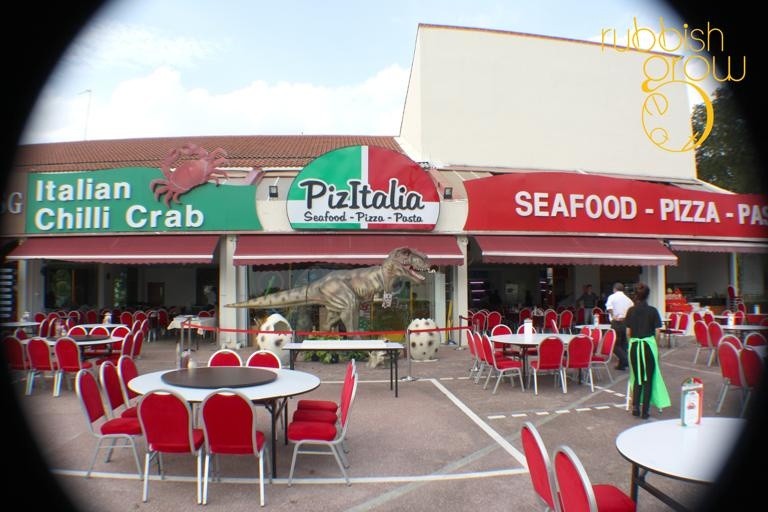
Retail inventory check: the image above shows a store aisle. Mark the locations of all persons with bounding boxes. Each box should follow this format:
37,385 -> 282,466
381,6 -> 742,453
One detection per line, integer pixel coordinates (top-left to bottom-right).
624,281 -> 672,419
605,281 -> 635,371
576,283 -> 598,325
207,286 -> 217,309
491,289 -> 503,305
524,289 -> 534,306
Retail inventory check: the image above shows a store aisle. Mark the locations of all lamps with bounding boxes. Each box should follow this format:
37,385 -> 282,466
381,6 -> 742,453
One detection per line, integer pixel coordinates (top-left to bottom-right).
444,187 -> 453,199
269,186 -> 278,197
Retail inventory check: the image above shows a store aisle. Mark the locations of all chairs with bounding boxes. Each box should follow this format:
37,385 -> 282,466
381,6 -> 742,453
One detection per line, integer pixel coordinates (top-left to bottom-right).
0,304 -> 358,506
466,285 -> 767,512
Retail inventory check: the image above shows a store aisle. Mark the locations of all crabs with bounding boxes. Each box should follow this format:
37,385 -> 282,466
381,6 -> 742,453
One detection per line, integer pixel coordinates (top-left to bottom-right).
151,141 -> 231,209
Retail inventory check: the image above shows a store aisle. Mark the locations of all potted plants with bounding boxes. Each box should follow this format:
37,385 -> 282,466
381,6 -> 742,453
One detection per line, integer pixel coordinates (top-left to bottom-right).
303,335 -> 401,363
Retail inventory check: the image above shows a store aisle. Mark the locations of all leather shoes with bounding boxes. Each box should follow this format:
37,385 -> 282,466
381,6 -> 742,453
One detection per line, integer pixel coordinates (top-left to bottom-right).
632,406 -> 650,419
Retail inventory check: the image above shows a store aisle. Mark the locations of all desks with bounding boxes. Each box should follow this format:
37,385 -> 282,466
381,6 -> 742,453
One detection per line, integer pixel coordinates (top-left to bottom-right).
281,339 -> 405,397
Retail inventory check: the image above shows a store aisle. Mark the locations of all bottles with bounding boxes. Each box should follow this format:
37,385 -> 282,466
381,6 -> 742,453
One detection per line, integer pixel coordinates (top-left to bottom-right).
683,390 -> 700,425
754,304 -> 760,314
727,313 -> 735,326
104,312 -> 112,324
593,314 -> 599,325
524,319 -> 533,336
